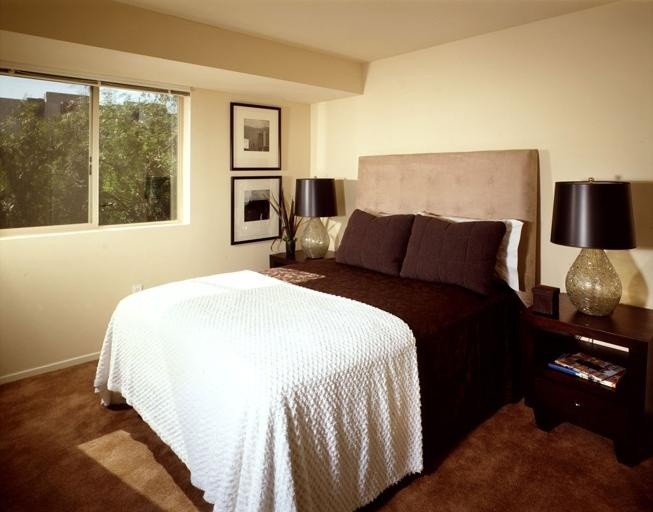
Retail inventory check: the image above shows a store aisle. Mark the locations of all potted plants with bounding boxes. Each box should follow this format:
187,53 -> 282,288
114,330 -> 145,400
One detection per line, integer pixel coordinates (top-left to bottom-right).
262,187 -> 297,260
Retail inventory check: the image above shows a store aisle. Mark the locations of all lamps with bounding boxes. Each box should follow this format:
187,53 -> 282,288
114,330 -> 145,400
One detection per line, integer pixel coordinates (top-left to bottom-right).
292,175 -> 340,260
549,174 -> 640,319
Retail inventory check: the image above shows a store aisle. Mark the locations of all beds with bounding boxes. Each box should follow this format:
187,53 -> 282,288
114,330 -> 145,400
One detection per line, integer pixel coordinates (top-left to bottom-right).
121,148 -> 539,509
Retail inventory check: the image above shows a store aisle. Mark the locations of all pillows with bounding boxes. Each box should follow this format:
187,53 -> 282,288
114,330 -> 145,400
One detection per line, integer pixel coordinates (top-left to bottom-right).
333,208 -> 524,297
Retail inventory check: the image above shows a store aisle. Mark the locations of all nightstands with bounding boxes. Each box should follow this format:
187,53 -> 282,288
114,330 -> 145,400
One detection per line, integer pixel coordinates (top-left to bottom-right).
520,291 -> 653,468
269,247 -> 339,272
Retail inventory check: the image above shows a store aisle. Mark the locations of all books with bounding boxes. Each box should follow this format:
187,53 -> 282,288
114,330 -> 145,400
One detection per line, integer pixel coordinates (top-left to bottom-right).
555,355 -> 624,381
549,356 -> 625,389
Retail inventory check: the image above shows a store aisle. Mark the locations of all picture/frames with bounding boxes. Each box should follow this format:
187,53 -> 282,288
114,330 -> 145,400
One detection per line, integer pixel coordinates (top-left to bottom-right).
230,102 -> 282,245
